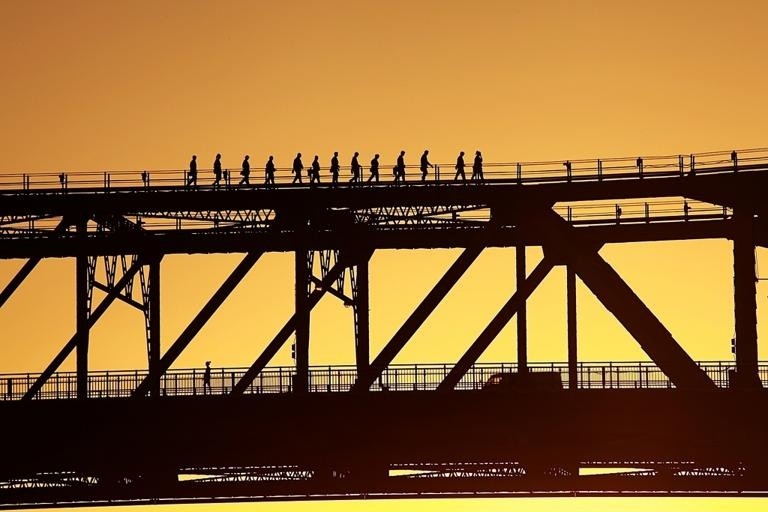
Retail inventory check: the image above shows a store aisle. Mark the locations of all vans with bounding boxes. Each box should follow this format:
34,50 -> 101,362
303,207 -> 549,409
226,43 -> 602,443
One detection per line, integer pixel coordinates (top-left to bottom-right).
480,372 -> 564,392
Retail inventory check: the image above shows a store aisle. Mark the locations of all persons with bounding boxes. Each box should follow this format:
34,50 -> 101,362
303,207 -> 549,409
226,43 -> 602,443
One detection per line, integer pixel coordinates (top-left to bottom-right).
238,155 -> 250,184
366,154 -> 380,181
454,151 -> 467,180
310,155 -> 320,183
329,152 -> 341,182
291,153 -> 304,183
471,150 -> 484,180
263,155 -> 277,184
211,153 -> 222,185
394,150 -> 406,181
420,150 -> 433,182
185,155 -> 197,186
201,360 -> 213,395
348,151 -> 363,182
